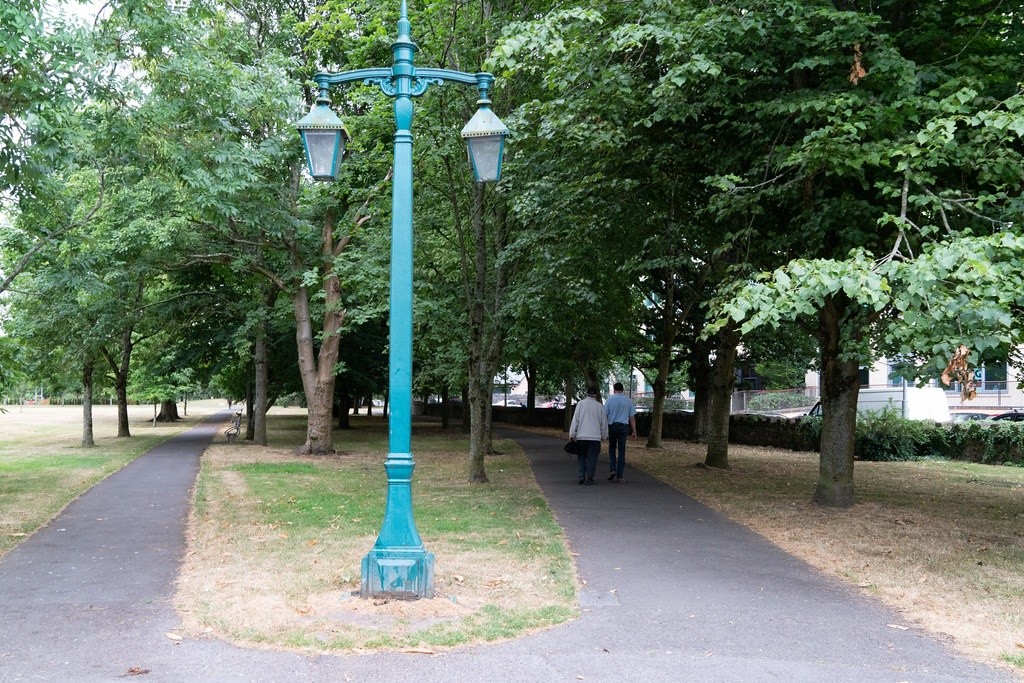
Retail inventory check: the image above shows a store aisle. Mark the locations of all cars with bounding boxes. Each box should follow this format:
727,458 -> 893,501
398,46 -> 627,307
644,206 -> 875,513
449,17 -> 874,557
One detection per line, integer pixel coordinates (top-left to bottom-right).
536,402 -> 566,410
961,408 -> 1024,423
491,400 -> 521,407
635,405 -> 650,413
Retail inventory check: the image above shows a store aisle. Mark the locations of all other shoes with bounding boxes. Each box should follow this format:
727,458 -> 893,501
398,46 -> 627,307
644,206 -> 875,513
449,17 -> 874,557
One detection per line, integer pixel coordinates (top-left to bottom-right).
584,477 -> 592,485
577,473 -> 585,485
615,478 -> 624,487
608,471 -> 616,480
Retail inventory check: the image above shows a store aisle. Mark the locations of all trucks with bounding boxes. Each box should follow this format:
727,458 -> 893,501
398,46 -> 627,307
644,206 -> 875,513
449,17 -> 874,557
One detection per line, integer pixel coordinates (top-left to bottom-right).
803,386 -> 951,425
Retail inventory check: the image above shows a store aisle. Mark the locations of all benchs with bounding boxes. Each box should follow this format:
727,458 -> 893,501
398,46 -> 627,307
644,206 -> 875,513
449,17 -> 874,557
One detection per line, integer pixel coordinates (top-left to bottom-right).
224,409 -> 242,443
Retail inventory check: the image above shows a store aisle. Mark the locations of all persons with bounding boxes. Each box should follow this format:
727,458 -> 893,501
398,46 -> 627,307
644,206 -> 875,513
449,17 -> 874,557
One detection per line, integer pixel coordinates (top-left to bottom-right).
568,386 -> 608,484
605,383 -> 637,484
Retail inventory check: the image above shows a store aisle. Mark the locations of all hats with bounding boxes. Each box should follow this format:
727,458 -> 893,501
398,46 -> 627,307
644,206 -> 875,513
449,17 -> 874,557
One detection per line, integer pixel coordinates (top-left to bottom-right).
586,386 -> 599,394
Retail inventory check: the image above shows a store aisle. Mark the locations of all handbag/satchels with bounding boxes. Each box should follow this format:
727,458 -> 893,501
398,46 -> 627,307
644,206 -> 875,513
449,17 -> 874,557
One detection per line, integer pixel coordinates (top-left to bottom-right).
564,438 -> 578,455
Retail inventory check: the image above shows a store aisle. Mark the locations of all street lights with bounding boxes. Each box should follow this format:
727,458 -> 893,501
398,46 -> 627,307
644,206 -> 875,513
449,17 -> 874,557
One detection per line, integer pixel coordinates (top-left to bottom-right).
293,1 -> 513,600
